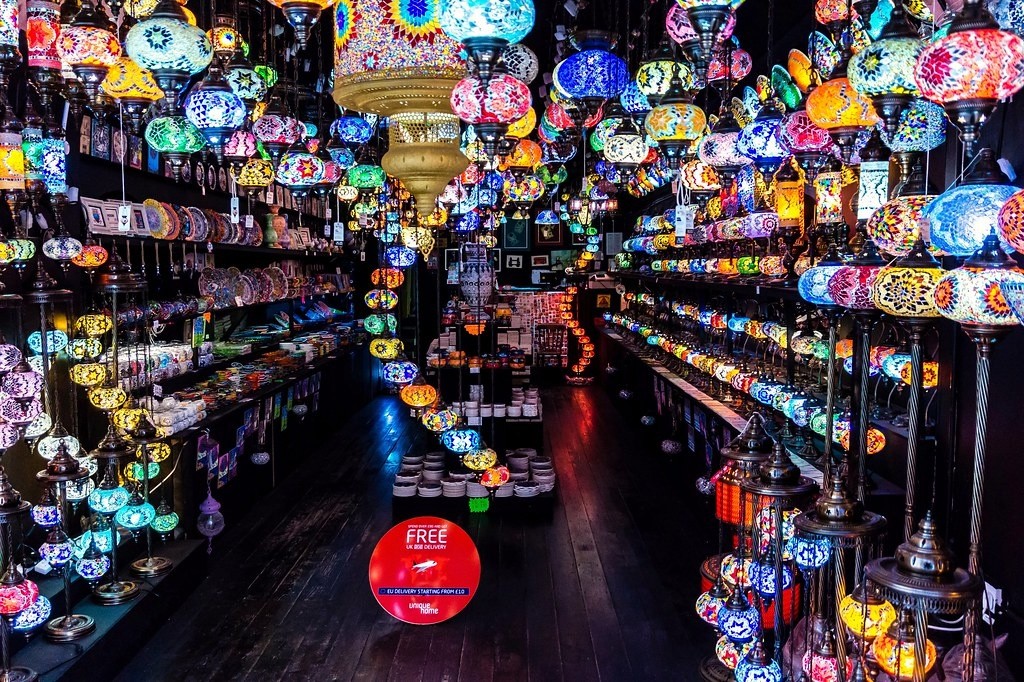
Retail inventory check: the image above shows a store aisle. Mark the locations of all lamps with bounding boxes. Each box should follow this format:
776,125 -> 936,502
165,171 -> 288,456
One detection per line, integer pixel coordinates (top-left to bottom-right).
0,0 -> 1024,682
